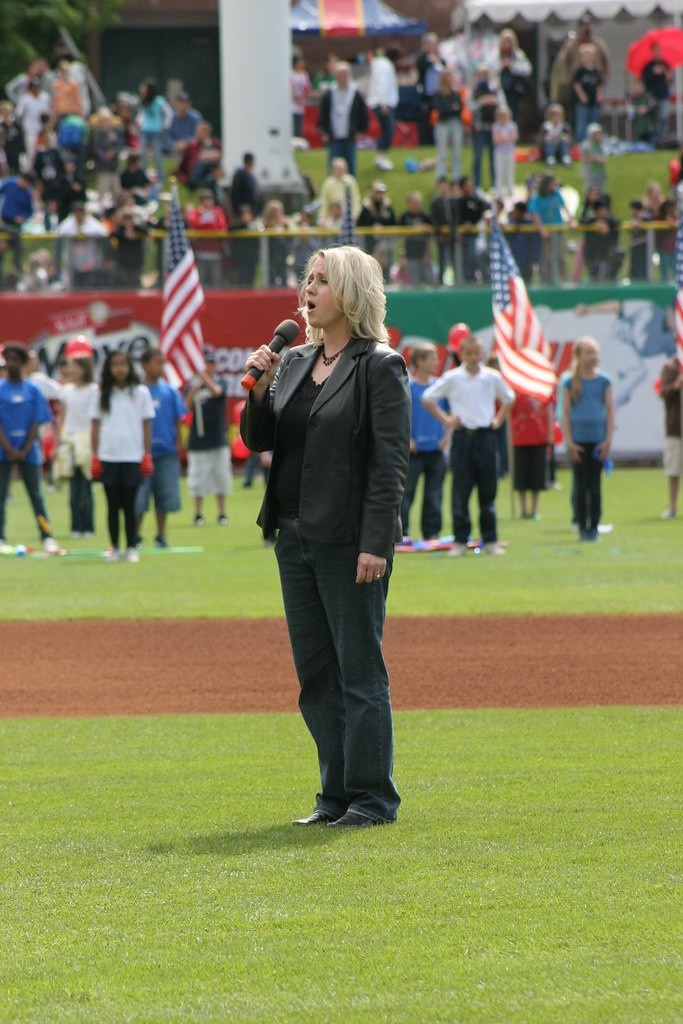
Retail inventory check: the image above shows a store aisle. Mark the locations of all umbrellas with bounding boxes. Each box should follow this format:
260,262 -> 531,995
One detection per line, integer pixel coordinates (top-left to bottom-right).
289,1 -> 428,38
628,25 -> 681,76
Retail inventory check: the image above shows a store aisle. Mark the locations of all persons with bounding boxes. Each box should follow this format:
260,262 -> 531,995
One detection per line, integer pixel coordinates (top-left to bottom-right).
0,45 -> 297,294
657,362 -> 681,521
237,246 -> 410,828
291,20 -> 673,284
560,337 -> 618,543
396,337 -> 516,557
0,341 -> 275,565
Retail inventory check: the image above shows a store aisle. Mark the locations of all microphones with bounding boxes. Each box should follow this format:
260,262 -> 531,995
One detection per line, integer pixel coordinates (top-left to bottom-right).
240,319 -> 299,391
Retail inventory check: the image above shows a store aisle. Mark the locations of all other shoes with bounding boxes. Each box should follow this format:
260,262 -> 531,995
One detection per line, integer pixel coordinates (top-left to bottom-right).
134,536 -> 143,548
42,536 -> 58,551
128,548 -> 138,562
292,812 -> 329,824
483,542 -> 504,556
329,809 -> 382,828
582,528 -> 599,542
156,535 -> 170,550
448,542 -> 467,556
112,548 -> 121,562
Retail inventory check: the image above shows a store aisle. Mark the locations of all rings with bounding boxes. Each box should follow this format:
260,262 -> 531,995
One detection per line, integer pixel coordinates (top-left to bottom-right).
374,574 -> 381,578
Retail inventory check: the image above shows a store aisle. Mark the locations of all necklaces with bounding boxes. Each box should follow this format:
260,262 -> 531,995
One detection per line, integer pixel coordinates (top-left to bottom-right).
320,344 -> 349,365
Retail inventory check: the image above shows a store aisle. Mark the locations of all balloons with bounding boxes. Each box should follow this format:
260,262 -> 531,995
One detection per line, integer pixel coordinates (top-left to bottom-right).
232,435 -> 251,458
233,401 -> 247,425
448,323 -> 473,351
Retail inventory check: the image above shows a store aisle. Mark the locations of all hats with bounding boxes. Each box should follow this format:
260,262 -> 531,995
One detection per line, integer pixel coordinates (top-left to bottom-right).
448,324 -> 470,351
204,346 -> 215,363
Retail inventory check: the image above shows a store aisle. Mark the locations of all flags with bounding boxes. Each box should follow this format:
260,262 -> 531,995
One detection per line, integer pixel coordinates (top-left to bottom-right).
675,183 -> 683,386
487,218 -> 557,405
157,195 -> 206,394
